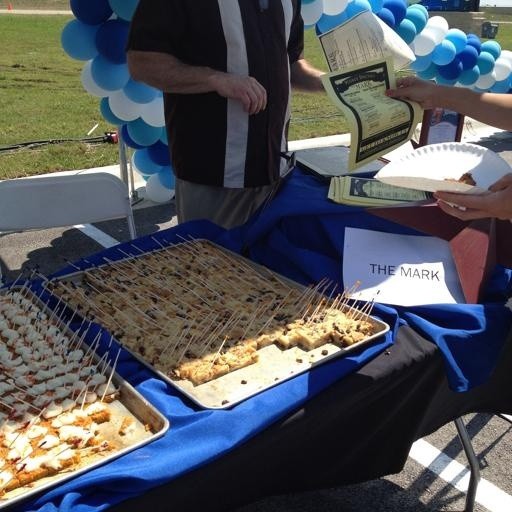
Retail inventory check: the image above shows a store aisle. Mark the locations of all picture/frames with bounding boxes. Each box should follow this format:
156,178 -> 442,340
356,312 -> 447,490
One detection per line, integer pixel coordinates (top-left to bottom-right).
419,107 -> 465,151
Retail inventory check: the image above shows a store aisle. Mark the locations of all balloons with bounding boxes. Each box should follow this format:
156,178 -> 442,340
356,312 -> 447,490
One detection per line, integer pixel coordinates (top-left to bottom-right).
59,0 -> 175,204
299,0 -> 511,94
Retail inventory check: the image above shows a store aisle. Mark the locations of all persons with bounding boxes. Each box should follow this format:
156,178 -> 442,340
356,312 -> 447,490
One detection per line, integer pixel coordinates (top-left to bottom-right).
385,75 -> 512,222
128,1 -> 331,231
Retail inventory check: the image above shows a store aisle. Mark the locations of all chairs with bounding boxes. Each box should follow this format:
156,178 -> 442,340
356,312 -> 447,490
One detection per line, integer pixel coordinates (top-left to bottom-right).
1,171 -> 136,240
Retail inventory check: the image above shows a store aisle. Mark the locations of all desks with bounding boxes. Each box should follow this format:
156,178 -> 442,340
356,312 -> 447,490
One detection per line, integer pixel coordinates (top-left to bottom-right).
1,154 -> 512,512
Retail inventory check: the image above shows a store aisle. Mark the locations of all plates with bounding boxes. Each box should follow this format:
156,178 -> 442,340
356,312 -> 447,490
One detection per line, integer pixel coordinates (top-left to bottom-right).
371,140 -> 511,195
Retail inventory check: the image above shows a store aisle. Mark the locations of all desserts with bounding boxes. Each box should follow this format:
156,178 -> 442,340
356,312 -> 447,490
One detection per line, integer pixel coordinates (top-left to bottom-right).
0,239 -> 375,502
